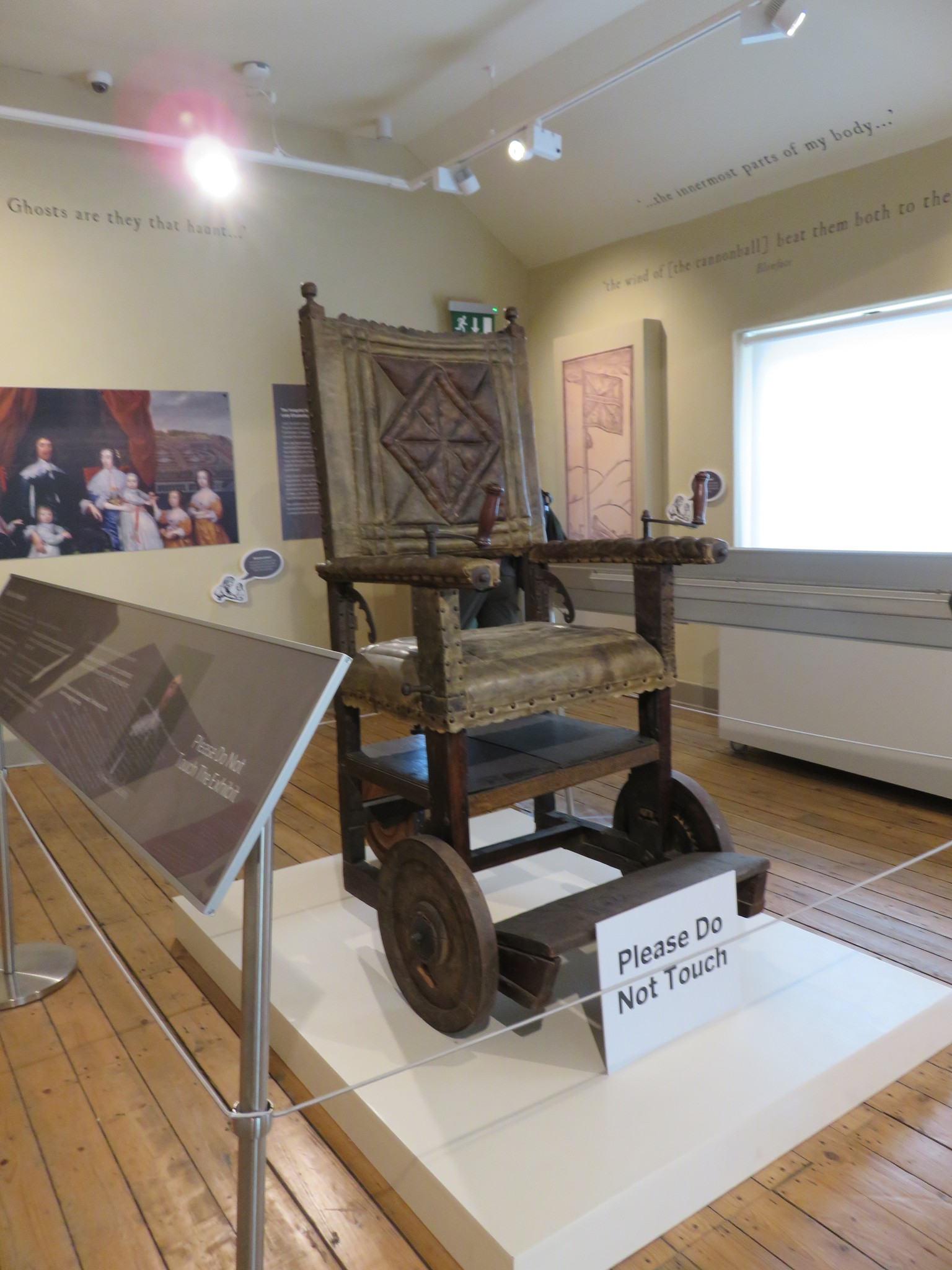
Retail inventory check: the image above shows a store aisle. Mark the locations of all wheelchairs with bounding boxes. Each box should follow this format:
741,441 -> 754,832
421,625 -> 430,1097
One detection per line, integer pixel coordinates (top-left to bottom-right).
297,275 -> 774,1040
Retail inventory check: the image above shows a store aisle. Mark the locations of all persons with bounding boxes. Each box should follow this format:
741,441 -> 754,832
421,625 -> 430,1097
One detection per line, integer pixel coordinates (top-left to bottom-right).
0,434 -> 232,560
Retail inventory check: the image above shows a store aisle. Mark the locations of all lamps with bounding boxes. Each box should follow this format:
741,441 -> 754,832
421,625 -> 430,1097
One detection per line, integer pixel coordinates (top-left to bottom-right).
739,0 -> 806,44
506,122 -> 562,162
433,162 -> 479,196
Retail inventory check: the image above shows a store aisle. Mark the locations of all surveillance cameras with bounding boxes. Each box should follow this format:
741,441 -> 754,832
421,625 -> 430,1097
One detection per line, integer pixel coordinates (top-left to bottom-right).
90,71 -> 113,93
